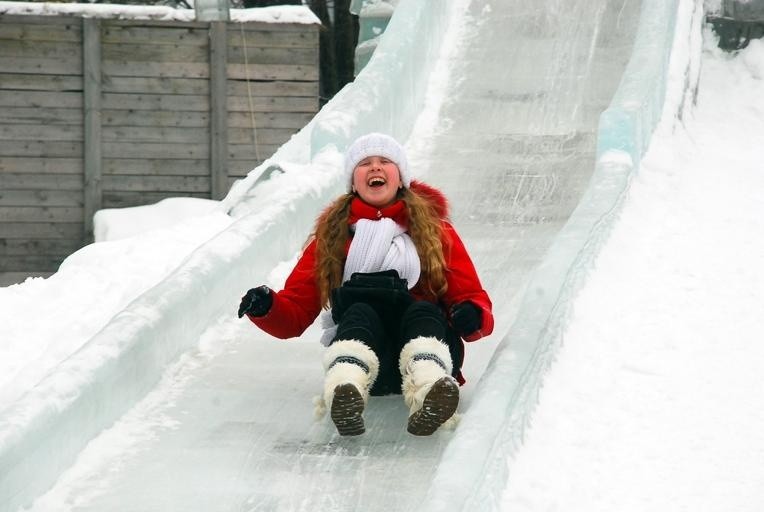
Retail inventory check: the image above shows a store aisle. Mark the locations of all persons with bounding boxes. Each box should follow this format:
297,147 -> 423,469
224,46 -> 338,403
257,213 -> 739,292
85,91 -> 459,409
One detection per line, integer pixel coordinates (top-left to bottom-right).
236,133 -> 494,436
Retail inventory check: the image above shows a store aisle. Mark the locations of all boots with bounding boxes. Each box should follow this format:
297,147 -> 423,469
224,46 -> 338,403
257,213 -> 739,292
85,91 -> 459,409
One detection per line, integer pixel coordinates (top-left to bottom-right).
321,339 -> 380,439
397,336 -> 461,438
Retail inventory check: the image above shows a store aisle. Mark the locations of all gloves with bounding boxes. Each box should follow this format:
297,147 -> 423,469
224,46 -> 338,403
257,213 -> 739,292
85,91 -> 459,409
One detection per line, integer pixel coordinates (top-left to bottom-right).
445,300 -> 483,338
235,284 -> 273,321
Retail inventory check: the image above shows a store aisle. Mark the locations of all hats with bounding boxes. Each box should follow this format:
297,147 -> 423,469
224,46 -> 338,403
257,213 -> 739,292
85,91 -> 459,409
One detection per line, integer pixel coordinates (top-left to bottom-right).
343,133 -> 411,195
